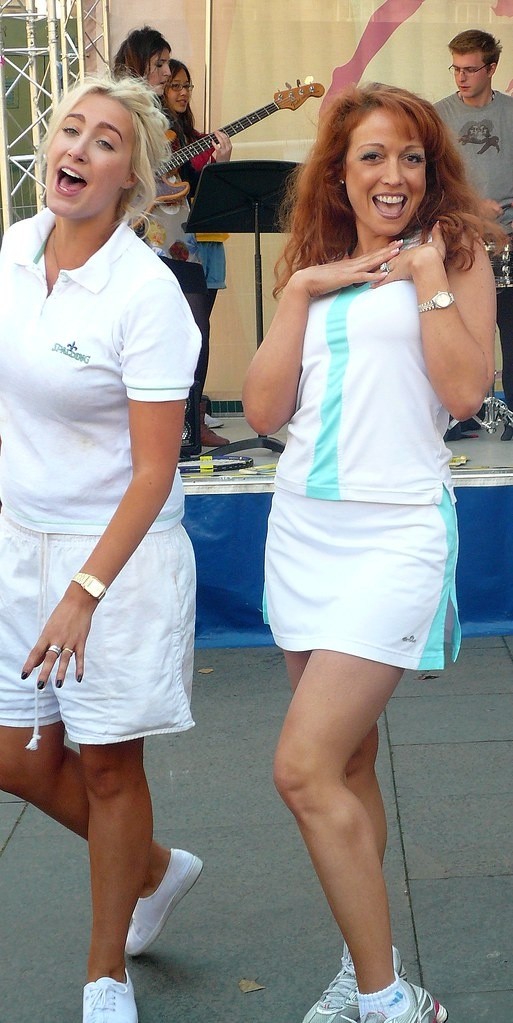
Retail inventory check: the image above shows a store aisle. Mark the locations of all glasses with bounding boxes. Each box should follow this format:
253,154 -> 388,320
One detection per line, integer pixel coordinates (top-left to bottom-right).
448,64 -> 486,77
170,84 -> 194,92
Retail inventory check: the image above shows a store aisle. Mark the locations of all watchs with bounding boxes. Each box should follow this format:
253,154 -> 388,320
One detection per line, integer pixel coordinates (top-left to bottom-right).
418,290 -> 455,312
71,573 -> 107,602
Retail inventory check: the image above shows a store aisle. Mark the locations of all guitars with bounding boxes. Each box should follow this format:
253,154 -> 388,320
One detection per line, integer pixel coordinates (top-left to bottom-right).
123,77 -> 328,224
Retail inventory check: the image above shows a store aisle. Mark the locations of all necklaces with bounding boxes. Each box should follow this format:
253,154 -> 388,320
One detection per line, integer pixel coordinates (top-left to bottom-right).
460,90 -> 495,102
53,233 -> 60,270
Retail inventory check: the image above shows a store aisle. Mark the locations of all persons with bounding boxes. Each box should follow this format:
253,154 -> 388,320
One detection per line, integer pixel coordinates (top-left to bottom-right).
113,28 -> 233,448
242,85 -> 502,1023
431,30 -> 513,440
0,73 -> 206,1023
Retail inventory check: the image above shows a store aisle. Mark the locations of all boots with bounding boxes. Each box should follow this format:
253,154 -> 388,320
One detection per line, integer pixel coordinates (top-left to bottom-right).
189,401 -> 230,447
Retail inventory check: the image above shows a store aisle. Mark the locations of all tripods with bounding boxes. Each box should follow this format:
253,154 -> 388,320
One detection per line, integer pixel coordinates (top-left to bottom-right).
181,159 -> 306,463
445,372 -> 513,437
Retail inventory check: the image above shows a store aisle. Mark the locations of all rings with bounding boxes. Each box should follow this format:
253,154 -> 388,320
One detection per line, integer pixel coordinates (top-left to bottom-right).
380,262 -> 391,274
63,648 -> 73,656
48,645 -> 61,657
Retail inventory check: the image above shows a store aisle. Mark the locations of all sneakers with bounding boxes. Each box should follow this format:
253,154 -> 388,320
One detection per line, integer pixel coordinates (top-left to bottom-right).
204,413 -> 224,428
125,848 -> 203,955
302,940 -> 449,1023
83,968 -> 139,1023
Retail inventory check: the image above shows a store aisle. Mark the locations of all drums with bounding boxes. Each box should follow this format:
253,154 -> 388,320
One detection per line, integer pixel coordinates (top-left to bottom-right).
482,238 -> 512,288
